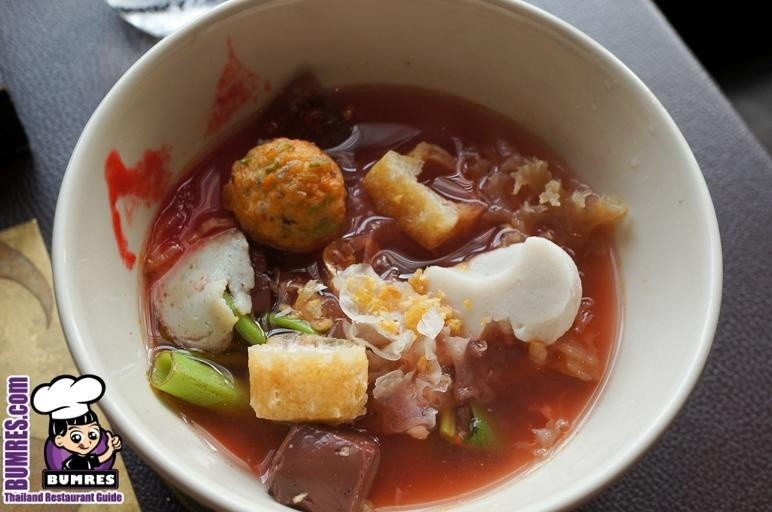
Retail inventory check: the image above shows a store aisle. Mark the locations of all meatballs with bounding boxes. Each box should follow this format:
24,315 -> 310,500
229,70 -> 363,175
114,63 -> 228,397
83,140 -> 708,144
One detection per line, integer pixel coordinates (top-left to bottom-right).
220,137 -> 347,252
